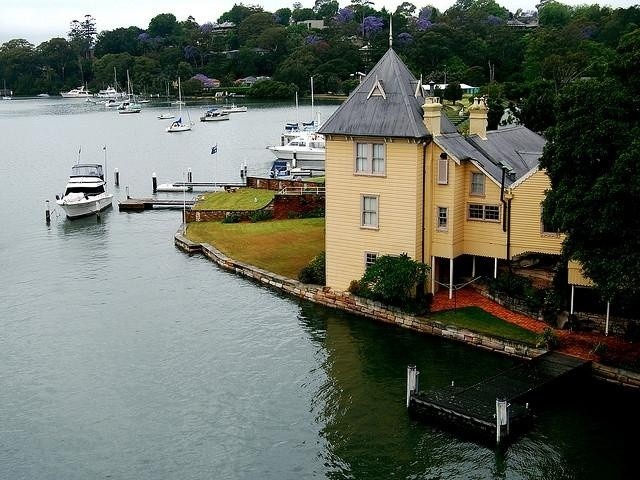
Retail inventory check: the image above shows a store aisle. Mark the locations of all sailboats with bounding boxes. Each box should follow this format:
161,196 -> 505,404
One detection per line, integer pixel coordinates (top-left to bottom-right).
93,66 -> 151,115
284,77 -> 324,138
166,76 -> 192,133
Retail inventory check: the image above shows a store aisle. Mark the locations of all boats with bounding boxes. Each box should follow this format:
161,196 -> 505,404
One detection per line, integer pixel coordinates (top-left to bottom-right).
86,98 -> 92,103
54,142 -> 113,220
157,91 -> 248,122
2,95 -> 14,100
60,81 -> 94,98
37,93 -> 50,97
266,134 -> 326,162
